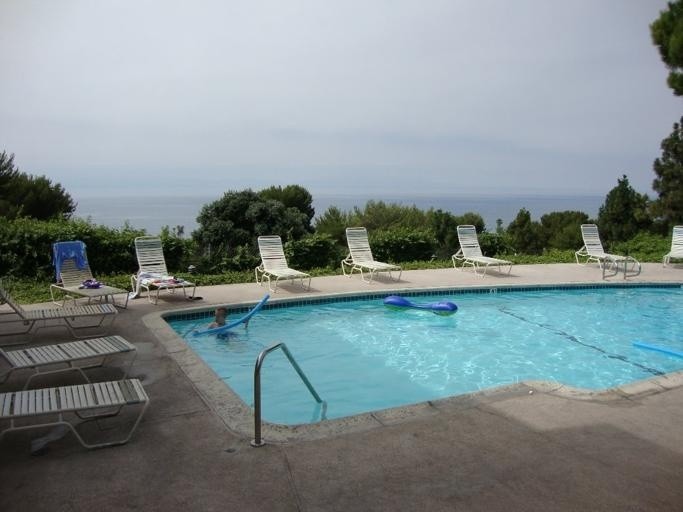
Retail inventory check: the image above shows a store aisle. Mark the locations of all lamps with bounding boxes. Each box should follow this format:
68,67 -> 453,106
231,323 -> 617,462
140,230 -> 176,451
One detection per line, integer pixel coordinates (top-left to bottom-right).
253,232 -> 312,293
574,223 -> 682,280
339,226 -> 403,283
45,235 -> 203,307
1,288 -> 149,452
450,224 -> 513,279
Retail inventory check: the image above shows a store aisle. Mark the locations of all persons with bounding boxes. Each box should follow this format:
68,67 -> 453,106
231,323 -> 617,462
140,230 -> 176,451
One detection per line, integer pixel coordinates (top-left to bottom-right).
193,307 -> 249,353
